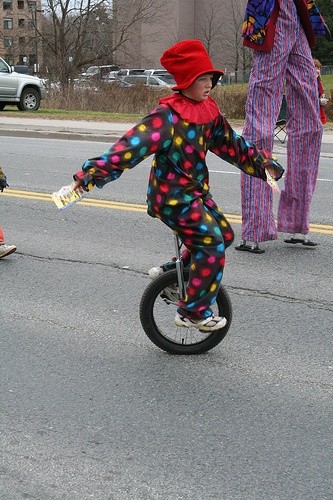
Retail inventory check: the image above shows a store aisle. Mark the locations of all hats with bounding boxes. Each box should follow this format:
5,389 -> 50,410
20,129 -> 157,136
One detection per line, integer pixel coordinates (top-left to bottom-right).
161,39 -> 225,92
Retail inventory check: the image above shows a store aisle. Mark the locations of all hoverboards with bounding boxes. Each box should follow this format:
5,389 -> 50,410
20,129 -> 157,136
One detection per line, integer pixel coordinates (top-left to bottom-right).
139,229 -> 232,356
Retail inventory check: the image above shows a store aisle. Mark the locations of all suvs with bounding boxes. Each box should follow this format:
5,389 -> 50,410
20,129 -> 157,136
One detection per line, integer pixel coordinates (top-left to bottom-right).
0,57 -> 46,111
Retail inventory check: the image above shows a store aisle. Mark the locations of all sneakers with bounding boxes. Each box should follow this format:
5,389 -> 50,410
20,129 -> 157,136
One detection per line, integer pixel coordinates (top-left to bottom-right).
0,245 -> 17,258
175,312 -> 227,332
149,260 -> 181,302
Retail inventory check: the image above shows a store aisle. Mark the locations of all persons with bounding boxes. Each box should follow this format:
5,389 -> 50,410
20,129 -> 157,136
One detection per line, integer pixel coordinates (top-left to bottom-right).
236,0 -> 328,253
0,169 -> 16,258
73,39 -> 285,333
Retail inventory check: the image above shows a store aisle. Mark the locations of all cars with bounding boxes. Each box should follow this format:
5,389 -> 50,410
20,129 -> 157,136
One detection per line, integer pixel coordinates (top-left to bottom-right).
79,64 -> 177,90
